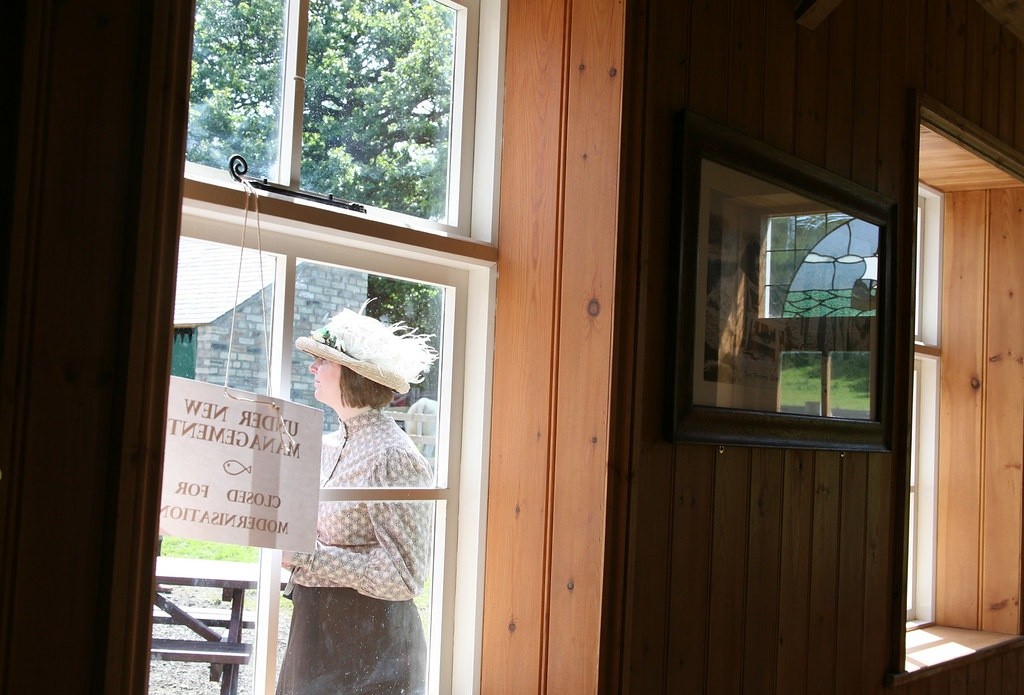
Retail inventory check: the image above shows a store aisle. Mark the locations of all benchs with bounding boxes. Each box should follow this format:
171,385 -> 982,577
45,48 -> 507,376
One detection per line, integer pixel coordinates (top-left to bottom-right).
150,606 -> 257,665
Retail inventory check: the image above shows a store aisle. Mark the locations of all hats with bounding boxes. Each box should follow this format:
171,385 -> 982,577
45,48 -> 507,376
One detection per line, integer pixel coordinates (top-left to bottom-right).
294,308 -> 438,394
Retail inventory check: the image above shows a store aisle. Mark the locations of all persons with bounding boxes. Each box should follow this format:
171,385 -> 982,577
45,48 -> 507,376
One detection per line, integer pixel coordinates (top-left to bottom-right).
274,308 -> 438,695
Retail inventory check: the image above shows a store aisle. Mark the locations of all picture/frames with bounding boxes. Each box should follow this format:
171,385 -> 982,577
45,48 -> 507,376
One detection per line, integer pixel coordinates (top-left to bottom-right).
669,109 -> 900,454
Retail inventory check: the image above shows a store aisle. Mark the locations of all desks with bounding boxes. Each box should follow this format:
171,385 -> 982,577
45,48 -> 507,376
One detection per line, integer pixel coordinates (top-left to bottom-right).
150,555 -> 293,695
